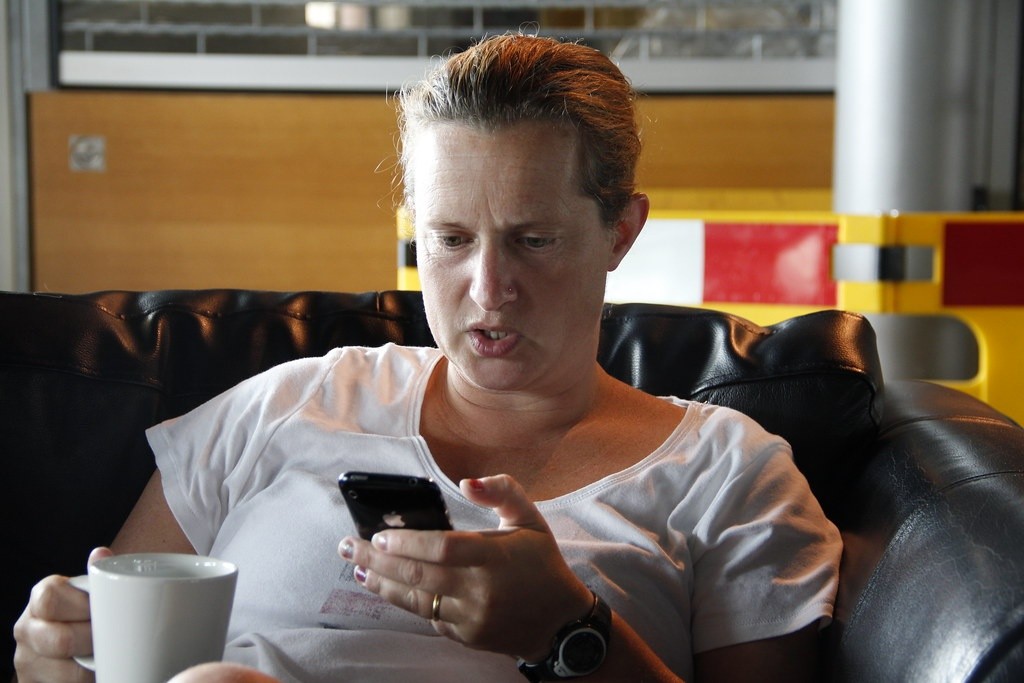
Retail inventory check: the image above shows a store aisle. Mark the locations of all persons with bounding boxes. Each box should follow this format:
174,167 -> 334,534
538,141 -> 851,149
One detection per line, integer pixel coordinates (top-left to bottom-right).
13,35 -> 843,683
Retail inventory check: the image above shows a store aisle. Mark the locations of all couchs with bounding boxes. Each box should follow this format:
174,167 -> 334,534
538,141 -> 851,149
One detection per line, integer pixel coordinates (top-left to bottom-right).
0,285 -> 1024,682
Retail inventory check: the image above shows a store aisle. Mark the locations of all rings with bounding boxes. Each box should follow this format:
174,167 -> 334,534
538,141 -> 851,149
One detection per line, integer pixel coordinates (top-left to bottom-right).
432,594 -> 441,621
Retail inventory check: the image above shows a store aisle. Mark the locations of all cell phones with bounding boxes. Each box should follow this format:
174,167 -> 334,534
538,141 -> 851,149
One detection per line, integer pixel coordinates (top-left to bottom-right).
339,471 -> 454,543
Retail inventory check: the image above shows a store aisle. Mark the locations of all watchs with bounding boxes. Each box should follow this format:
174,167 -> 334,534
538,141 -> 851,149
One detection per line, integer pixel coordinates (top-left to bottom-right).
518,589 -> 612,683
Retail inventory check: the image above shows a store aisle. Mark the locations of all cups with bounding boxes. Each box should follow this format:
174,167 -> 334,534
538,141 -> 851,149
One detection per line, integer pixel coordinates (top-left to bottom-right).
66,552 -> 239,683
